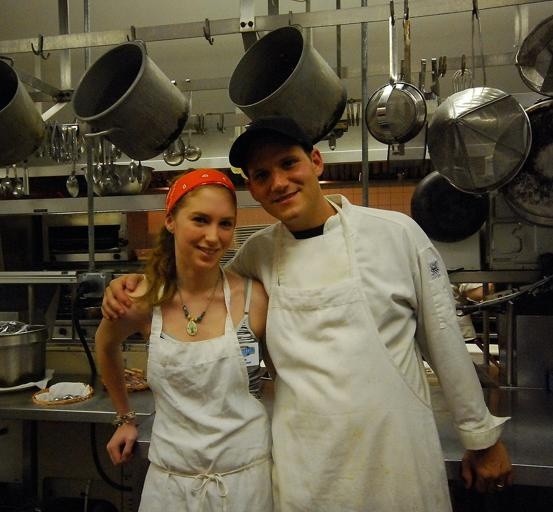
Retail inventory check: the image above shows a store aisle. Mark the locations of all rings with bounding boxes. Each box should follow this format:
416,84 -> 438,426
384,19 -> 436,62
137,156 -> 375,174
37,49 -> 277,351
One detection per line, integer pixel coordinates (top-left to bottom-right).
495,481 -> 508,491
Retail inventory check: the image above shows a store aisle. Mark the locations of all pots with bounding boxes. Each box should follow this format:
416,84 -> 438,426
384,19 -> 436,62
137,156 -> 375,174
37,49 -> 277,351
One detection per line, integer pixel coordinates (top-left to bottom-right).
0,322 -> 48,386
226,25 -> 347,145
411,168 -> 492,244
1,54 -> 47,168
71,38 -> 191,160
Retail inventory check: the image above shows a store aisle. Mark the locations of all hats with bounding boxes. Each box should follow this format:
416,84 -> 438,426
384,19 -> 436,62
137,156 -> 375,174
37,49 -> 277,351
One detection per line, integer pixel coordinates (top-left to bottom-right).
228,114 -> 313,168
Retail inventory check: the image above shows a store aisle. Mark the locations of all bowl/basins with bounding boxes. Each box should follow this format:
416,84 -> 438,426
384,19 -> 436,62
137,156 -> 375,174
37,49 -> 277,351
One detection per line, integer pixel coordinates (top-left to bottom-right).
82,164 -> 155,196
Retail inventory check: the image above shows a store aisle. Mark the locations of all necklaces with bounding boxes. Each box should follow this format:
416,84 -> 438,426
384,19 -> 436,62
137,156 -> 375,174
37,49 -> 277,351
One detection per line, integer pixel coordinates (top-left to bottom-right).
172,269 -> 221,338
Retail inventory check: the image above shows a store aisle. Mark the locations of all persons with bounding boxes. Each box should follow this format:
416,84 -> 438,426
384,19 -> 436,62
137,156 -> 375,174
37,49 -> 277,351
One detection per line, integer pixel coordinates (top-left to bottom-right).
91,166 -> 280,512
97,115 -> 516,511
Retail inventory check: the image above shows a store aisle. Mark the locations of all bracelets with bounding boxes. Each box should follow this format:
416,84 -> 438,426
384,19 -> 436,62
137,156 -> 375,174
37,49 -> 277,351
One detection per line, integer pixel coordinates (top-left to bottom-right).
110,408 -> 137,428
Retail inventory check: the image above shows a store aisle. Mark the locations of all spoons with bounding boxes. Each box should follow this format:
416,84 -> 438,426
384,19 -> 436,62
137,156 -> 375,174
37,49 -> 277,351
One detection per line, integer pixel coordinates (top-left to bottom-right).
65,163 -> 80,199
